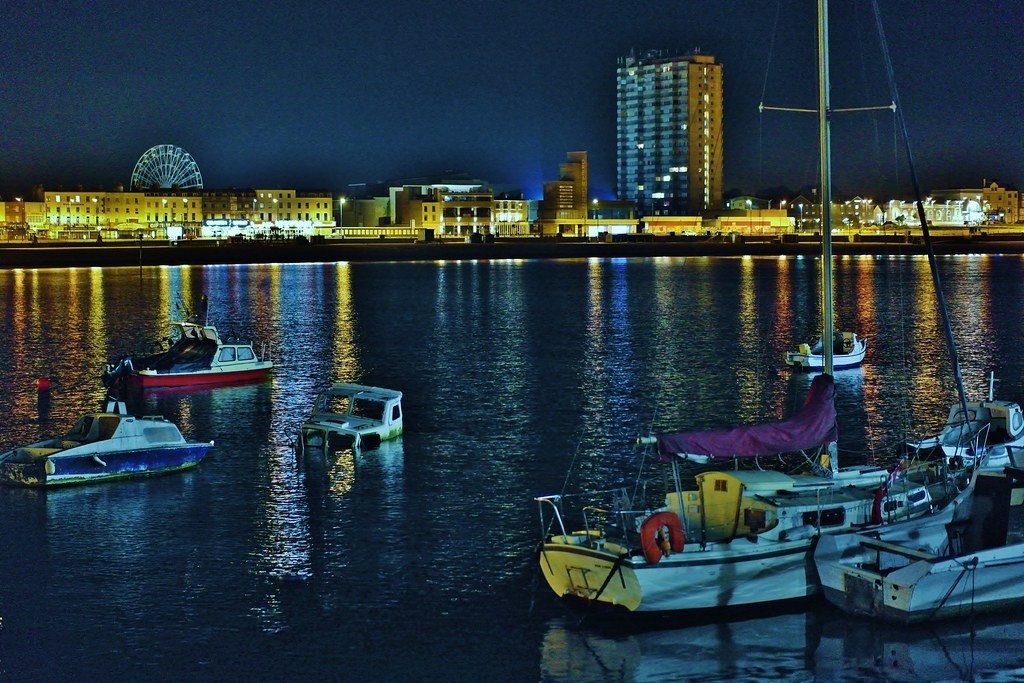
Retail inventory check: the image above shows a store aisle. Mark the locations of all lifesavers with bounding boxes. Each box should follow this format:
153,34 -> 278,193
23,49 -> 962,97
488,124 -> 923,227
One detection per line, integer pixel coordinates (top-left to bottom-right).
640,510 -> 684,566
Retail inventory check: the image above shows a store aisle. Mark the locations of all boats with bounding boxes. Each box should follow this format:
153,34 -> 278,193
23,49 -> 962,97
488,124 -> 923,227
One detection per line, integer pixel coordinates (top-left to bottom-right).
906,371 -> 1024,469
782,330 -> 867,370
0,396 -> 214,485
101,291 -> 273,387
818,423 -> 1022,622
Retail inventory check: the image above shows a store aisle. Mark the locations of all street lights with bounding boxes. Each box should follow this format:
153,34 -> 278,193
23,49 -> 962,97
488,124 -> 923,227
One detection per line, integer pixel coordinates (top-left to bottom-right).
799,204 -> 804,233
779,200 -> 787,240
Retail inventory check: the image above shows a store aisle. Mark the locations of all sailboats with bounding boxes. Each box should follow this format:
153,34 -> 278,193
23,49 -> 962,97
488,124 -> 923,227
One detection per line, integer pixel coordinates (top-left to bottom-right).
539,0 -> 995,612
